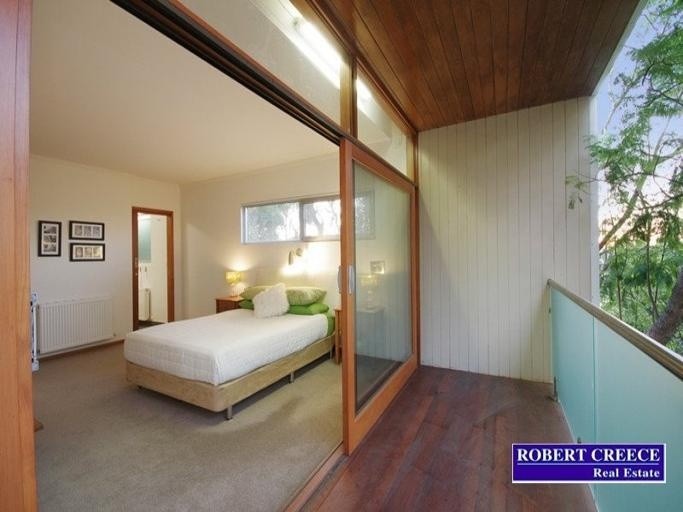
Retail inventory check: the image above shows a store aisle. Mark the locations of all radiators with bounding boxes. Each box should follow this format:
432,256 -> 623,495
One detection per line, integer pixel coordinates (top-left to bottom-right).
138,288 -> 150,321
39,297 -> 113,354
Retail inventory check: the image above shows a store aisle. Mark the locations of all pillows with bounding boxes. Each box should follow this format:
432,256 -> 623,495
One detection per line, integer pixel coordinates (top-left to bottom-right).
252,283 -> 289,318
239,286 -> 326,306
239,300 -> 329,315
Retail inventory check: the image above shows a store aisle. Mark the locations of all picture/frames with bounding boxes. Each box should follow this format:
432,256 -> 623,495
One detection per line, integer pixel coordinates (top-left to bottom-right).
69,243 -> 105,261
38,220 -> 61,257
68,220 -> 104,240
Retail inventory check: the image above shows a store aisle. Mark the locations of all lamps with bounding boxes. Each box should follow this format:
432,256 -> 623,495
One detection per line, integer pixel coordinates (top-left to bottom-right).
358,275 -> 377,310
226,272 -> 239,298
288,250 -> 293,264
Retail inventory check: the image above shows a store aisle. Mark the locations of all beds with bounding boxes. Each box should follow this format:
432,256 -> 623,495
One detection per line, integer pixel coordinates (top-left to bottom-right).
124,307 -> 335,419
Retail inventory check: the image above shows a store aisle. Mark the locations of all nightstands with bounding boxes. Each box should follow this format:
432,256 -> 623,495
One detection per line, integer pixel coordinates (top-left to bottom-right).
215,296 -> 242,313
334,306 -> 385,365
